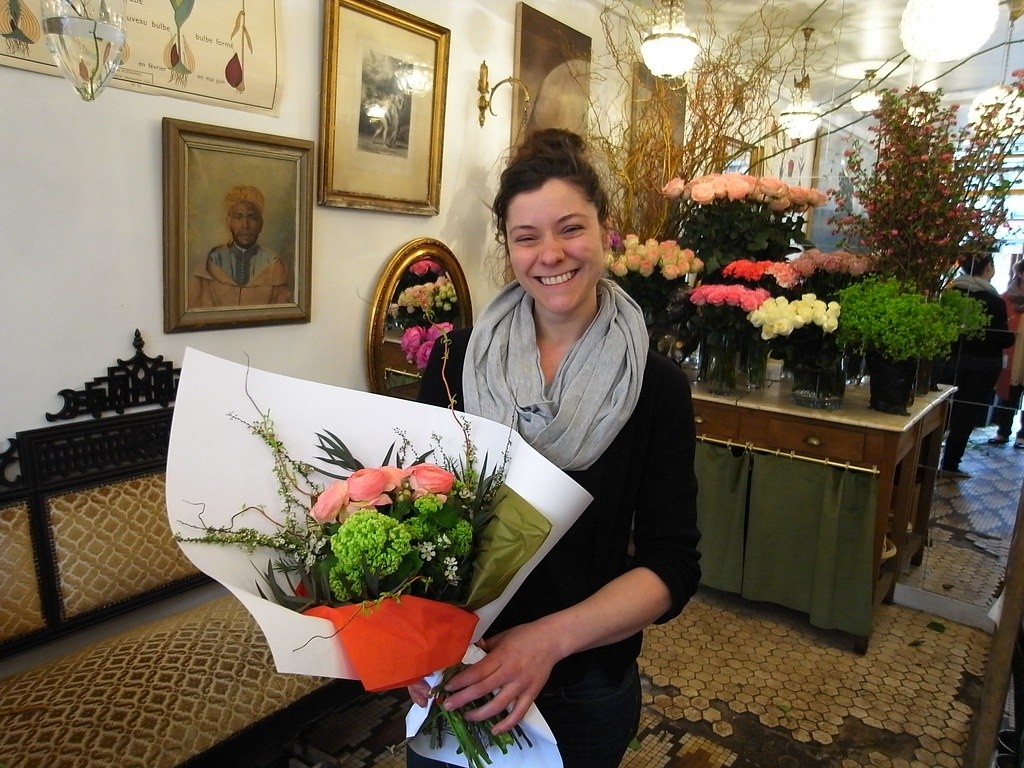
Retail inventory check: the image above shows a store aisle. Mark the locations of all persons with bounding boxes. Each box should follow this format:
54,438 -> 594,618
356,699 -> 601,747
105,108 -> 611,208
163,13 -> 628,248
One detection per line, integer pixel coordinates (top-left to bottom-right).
404,128 -> 702,768
937,252 -> 1024,478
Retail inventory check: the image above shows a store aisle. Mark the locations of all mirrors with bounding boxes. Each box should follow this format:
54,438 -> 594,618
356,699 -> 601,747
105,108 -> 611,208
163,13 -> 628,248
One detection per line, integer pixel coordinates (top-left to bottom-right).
367,237 -> 475,403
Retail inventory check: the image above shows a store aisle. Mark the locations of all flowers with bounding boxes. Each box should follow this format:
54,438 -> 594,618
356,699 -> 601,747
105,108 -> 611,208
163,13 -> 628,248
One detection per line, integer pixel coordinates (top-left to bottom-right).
164,345 -> 596,768
386,261 -> 458,370
600,172 -> 877,396
826,68 -> 1023,300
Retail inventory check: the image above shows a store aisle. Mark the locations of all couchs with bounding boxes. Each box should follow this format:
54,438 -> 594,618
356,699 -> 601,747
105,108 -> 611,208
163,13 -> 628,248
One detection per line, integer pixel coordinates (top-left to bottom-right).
0,328 -> 362,768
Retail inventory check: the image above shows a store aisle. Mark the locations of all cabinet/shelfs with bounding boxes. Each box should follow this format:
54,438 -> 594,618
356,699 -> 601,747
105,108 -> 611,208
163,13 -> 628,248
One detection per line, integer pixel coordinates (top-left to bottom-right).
674,362 -> 934,655
866,376 -> 960,584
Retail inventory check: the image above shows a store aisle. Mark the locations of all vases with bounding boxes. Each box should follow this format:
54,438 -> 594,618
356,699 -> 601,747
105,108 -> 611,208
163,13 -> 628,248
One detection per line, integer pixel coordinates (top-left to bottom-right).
659,331 -> 866,410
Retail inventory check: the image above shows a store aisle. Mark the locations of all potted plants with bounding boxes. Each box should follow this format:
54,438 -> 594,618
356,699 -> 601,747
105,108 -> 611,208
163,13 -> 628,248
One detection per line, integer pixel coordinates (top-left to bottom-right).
837,274 -> 994,417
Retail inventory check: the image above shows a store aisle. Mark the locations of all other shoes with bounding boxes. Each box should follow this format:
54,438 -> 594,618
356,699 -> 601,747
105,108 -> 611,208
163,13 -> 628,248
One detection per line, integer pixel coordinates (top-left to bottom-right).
988,437 -> 1010,445
995,754 -> 1020,768
998,729 -> 1015,753
1014,441 -> 1024,449
940,466 -> 969,479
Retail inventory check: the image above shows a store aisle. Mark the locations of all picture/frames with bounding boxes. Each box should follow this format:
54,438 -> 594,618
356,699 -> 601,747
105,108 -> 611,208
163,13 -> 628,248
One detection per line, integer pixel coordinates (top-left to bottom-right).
715,134 -> 759,179
315,0 -> 452,217
161,116 -> 316,334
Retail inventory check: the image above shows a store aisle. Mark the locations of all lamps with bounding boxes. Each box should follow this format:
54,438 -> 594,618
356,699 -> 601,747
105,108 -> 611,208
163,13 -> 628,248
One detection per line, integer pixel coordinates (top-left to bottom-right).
639,0 -> 700,81
778,26 -> 821,139
968,0 -> 1024,138
851,69 -> 882,113
